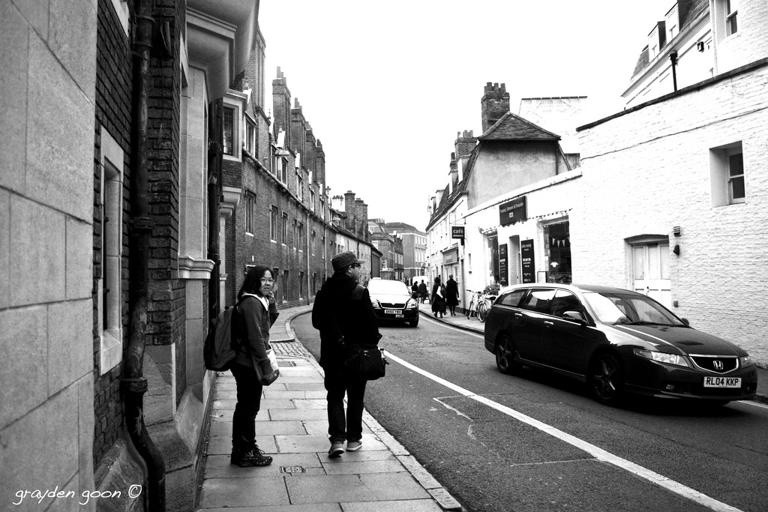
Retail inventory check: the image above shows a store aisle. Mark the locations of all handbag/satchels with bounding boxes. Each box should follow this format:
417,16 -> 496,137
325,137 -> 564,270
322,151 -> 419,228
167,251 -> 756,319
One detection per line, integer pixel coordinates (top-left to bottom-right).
338,334 -> 386,380
252,349 -> 280,385
203,306 -> 237,371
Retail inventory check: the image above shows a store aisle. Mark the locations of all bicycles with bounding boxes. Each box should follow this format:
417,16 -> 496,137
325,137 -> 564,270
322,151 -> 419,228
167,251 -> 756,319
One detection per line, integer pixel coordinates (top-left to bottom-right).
463,288 -> 495,324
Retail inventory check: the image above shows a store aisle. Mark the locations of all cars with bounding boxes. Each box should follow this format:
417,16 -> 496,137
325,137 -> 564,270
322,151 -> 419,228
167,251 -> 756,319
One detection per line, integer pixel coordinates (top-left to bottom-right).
475,282 -> 757,411
364,277 -> 419,330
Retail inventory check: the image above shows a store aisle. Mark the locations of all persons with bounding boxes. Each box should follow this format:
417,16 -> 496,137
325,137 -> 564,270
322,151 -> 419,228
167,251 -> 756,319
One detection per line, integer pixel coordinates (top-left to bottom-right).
230,266 -> 280,467
312,252 -> 389,456
404,274 -> 459,318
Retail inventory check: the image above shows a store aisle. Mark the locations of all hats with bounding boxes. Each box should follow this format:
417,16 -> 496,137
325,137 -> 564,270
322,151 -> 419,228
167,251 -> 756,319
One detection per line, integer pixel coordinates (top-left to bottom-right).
333,251 -> 365,270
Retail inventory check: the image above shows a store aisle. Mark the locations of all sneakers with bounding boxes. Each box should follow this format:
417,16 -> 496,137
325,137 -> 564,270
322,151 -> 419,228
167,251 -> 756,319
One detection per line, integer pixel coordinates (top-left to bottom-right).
231,448 -> 272,467
328,441 -> 344,458
346,441 -> 362,451
